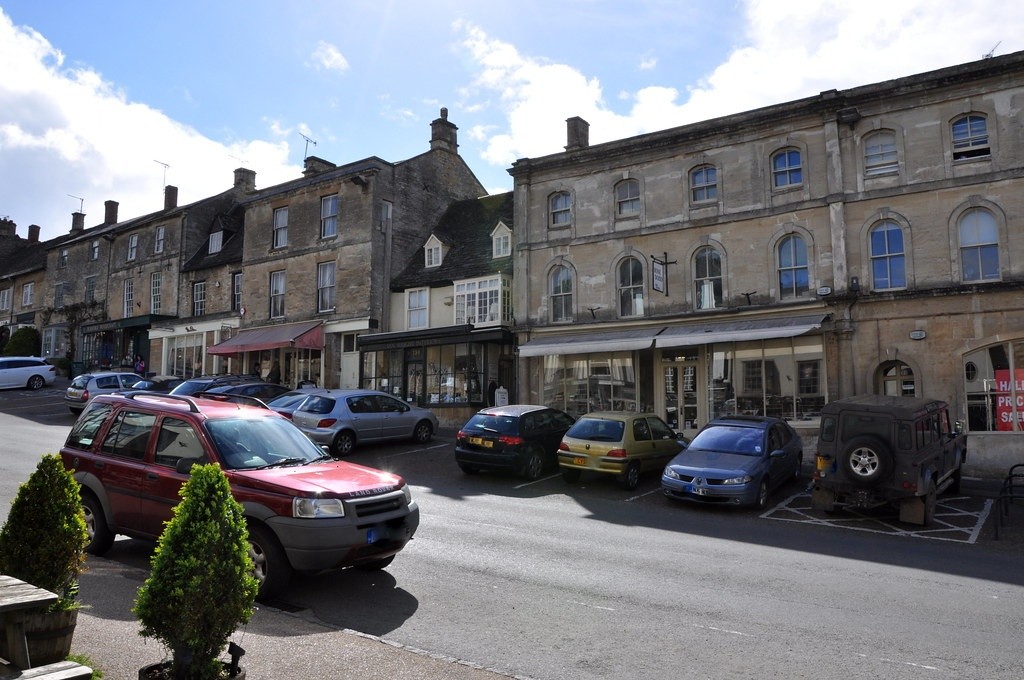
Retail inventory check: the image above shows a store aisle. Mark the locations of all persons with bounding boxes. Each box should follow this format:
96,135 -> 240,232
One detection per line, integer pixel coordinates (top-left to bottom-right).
251,361 -> 282,384
134,354 -> 147,377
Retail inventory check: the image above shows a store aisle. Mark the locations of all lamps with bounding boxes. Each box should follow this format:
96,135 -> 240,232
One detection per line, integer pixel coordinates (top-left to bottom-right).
102,233 -> 115,242
836,106 -> 862,131
350,175 -> 369,186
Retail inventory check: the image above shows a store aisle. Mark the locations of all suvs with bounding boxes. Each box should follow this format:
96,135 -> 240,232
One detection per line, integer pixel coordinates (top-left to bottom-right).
169,373 -> 267,396
811,394 -> 969,529
60,390 -> 420,604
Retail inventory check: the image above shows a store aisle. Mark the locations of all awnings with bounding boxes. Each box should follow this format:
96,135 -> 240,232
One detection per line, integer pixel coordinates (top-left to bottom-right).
516,315 -> 832,356
206,320 -> 323,355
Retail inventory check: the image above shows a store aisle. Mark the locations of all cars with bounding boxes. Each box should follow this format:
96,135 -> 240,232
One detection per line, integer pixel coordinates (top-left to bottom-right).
291,388 -> 439,456
64,371 -> 144,415
454,404 -> 577,480
660,415 -> 803,511
132,375 -> 186,394
206,382 -> 290,403
557,411 -> 693,490
264,387 -> 328,422
0,354 -> 57,390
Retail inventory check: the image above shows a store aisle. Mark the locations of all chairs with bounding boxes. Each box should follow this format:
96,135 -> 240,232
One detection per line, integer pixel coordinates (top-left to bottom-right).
215,428 -> 252,459
